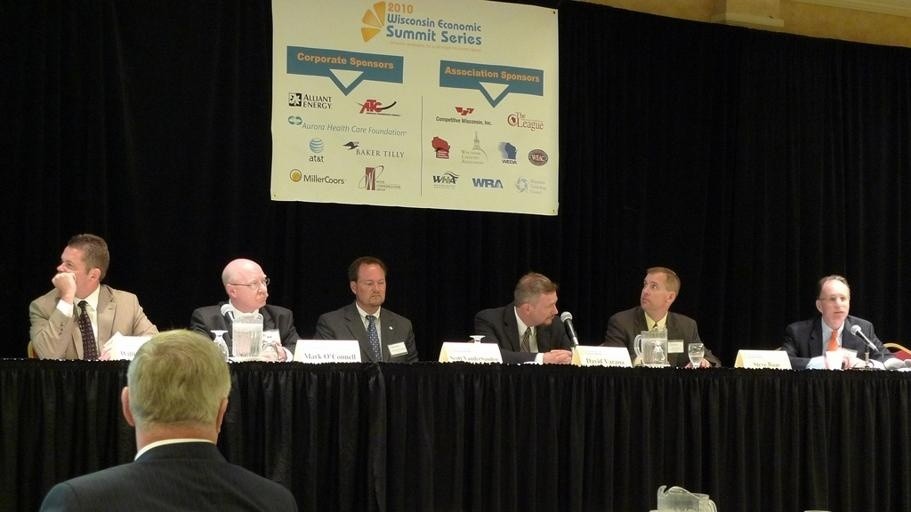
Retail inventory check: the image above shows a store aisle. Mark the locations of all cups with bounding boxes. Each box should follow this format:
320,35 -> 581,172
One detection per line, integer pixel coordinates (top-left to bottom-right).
470,335 -> 486,343
232,313 -> 264,363
633,330 -> 668,367
211,330 -> 229,361
826,350 -> 850,370
688,342 -> 705,368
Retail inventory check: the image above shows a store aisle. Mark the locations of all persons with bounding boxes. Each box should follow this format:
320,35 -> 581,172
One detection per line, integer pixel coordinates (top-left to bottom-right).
779,274 -> 902,369
475,273 -> 580,364
313,257 -> 420,363
40,330 -> 299,512
30,233 -> 161,361
192,259 -> 303,363
606,268 -> 723,368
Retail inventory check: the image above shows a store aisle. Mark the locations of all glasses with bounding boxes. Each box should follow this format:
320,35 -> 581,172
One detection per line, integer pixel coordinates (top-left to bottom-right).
229,276 -> 271,288
821,295 -> 849,305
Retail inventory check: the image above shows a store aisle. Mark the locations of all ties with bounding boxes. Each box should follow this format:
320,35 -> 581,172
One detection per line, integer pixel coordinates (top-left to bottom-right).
365,314 -> 382,361
521,328 -> 535,354
75,299 -> 98,361
827,330 -> 840,351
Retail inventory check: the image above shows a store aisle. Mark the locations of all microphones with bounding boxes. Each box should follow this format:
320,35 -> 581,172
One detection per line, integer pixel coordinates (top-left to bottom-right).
561,311 -> 581,347
849,324 -> 882,352
219,304 -> 236,323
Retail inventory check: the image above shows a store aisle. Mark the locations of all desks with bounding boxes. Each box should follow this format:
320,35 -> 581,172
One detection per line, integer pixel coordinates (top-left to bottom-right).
0,357 -> 911,512
